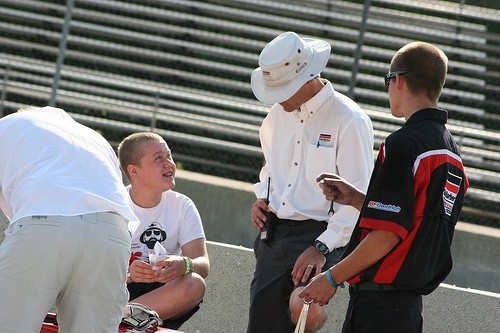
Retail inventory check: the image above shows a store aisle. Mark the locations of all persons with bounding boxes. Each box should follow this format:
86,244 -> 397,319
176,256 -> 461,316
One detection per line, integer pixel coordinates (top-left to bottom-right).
298,42 -> 470,333
241,31 -> 374,333
0,107 -> 138,333
117,132 -> 210,333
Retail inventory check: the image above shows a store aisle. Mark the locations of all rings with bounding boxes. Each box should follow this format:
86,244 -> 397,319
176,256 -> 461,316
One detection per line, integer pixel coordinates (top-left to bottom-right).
307,264 -> 313,268
302,296 -> 314,305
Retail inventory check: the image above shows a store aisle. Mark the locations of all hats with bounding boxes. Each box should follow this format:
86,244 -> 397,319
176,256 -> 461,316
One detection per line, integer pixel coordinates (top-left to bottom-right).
251,30 -> 331,103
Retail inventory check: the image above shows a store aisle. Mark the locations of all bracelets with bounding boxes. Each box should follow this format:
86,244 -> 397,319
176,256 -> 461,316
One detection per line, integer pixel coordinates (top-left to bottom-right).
127,272 -> 134,283
184,255 -> 194,275
326,269 -> 345,288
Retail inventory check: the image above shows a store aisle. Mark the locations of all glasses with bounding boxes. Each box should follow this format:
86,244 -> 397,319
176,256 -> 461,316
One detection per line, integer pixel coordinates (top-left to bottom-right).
385,70 -> 409,86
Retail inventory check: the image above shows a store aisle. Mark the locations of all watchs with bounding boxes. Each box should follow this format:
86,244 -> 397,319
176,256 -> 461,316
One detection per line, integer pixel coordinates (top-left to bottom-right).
314,240 -> 329,256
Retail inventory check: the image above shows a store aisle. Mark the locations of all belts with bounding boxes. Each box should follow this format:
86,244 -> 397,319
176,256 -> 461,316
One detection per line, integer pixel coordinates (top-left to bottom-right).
352,282 -> 408,291
96,212 -> 128,229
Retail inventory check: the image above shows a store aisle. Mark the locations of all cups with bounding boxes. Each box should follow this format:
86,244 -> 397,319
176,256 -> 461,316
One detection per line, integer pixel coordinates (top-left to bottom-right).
148,250 -> 166,276
